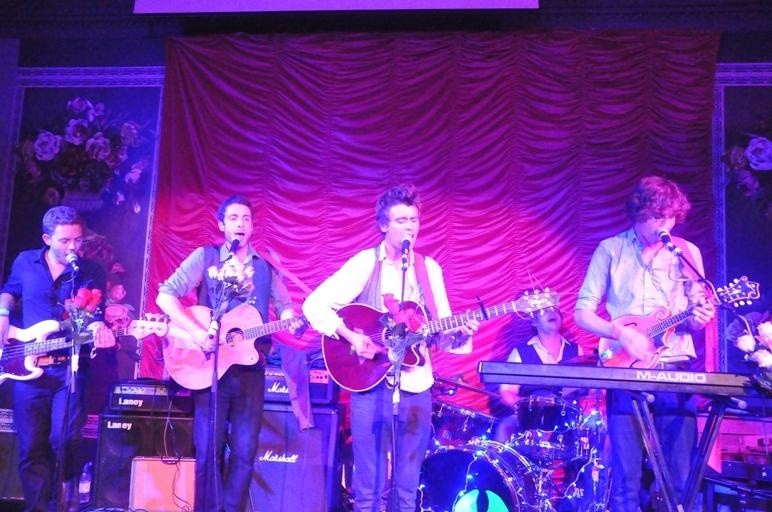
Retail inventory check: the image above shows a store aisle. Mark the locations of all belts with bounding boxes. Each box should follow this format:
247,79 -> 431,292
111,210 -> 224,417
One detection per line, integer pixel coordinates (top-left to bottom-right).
36,355 -> 71,366
654,361 -> 685,371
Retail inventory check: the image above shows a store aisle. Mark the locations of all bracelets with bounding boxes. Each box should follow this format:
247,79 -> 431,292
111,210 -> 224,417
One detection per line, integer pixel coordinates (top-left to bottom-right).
0,308 -> 10,316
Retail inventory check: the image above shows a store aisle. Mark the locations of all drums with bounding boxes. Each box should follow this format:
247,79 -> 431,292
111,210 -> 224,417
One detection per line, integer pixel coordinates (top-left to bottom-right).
414,438 -> 544,511
432,395 -> 501,449
507,426 -> 607,465
516,393 -> 582,430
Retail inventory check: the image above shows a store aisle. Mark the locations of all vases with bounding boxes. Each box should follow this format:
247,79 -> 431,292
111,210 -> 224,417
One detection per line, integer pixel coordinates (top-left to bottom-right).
61,184 -> 102,227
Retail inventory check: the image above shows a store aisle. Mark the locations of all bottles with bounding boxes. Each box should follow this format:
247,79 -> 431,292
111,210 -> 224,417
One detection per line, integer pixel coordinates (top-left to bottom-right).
77,463 -> 93,503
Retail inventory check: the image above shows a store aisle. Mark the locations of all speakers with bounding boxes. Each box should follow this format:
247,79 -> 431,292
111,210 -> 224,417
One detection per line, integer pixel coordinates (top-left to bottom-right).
244,401 -> 349,512
89,409 -> 196,511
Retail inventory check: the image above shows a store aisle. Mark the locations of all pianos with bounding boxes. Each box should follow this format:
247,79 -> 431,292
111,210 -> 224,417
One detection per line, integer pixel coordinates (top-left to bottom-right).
470,354 -> 749,407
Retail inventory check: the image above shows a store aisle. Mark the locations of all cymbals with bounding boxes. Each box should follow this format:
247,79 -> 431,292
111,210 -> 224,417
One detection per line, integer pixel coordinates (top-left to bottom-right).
432,372 -> 503,400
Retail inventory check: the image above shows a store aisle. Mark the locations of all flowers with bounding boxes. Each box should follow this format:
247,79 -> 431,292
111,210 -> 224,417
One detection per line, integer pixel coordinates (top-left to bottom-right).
104,261 -> 134,330
15,95 -> 149,213
64,287 -> 103,372
381,293 -> 425,416
724,311 -> 772,392
205,255 -> 257,338
720,133 -> 772,219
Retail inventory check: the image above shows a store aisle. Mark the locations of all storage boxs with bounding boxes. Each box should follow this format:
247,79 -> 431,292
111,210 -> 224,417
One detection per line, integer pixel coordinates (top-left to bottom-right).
128,456 -> 196,512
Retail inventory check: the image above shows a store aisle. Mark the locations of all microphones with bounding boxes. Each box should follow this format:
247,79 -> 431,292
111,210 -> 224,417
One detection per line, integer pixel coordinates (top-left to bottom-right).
66,253 -> 80,272
228,238 -> 240,256
655,229 -> 683,256
401,239 -> 410,270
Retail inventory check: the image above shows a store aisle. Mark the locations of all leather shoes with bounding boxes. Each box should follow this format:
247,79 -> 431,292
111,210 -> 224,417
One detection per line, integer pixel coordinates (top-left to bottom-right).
60,477 -> 80,512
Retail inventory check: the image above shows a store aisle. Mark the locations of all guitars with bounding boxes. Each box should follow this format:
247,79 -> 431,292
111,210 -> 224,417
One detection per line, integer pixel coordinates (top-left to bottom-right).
598,276 -> 761,369
319,285 -> 562,395
0,315 -> 171,385
158,301 -> 311,396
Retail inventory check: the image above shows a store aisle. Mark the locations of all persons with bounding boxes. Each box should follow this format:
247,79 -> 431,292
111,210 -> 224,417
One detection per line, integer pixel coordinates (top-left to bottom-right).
575,175 -> 714,511
154,193 -> 308,511
0,205 -> 114,512
494,305 -> 590,445
302,181 -> 479,511
